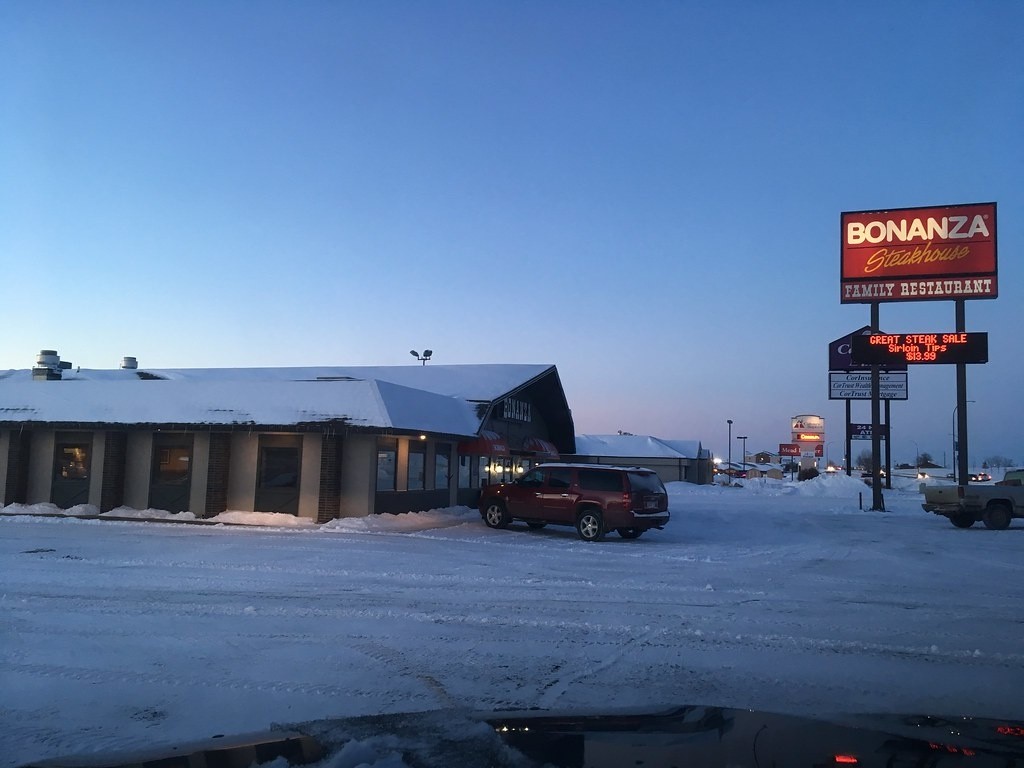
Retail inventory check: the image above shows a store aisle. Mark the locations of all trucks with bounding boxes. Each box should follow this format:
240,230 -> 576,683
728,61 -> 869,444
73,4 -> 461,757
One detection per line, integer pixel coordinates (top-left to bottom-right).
921,469 -> 1024,530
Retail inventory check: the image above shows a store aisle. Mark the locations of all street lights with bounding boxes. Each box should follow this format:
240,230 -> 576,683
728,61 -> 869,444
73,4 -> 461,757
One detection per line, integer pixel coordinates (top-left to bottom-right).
952,400 -> 975,482
726,419 -> 733,484
736,436 -> 747,472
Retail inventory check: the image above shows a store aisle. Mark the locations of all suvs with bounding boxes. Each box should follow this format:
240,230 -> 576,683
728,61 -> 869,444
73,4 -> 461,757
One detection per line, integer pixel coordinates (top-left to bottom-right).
477,462 -> 671,542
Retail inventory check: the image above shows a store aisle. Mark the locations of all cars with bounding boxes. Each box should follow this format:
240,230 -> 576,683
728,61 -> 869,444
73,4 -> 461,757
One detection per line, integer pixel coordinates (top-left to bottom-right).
976,472 -> 990,482
946,473 -> 954,478
917,473 -> 928,479
968,473 -> 978,482
862,468 -> 887,478
